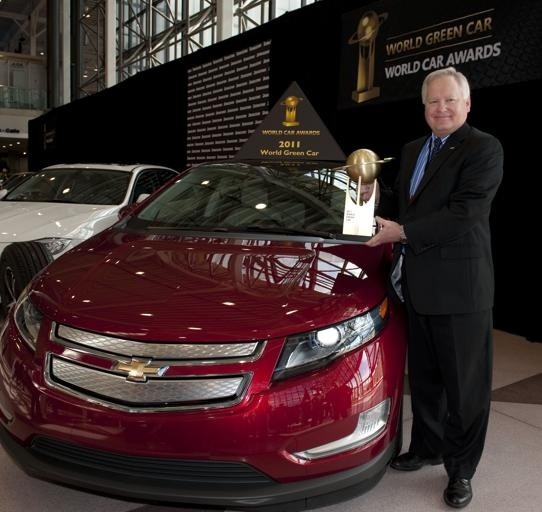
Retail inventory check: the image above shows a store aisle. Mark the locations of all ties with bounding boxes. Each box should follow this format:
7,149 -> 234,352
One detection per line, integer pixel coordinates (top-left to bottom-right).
430,137 -> 442,161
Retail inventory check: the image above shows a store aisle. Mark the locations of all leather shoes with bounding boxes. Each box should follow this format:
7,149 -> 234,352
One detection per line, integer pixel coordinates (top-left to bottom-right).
444,478 -> 473,508
390,451 -> 443,471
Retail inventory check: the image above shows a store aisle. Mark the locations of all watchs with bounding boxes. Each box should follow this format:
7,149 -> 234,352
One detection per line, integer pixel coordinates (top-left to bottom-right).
400,224 -> 408,245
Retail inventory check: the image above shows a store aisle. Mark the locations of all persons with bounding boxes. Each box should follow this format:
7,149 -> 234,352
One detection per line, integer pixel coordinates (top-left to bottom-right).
365,66 -> 503,508
